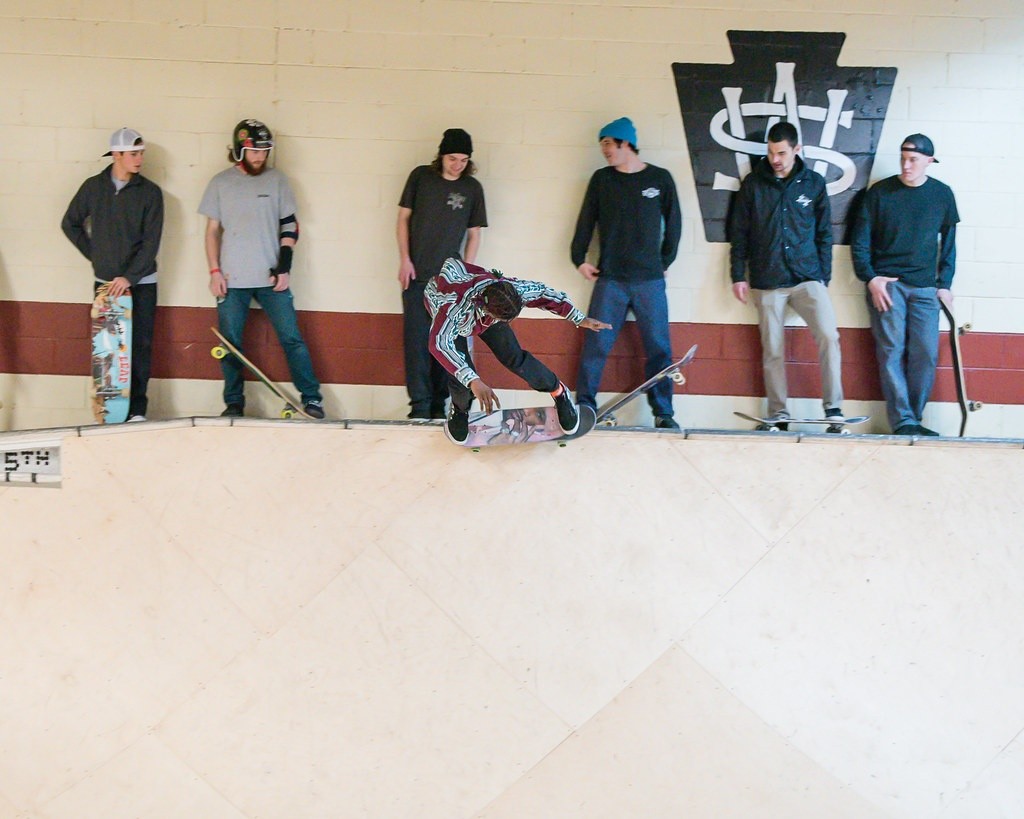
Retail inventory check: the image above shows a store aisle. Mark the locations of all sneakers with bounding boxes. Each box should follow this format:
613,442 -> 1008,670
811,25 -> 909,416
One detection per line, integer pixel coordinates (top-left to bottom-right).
443,402 -> 470,445
655,414 -> 678,428
550,381 -> 580,435
221,405 -> 243,416
305,400 -> 324,419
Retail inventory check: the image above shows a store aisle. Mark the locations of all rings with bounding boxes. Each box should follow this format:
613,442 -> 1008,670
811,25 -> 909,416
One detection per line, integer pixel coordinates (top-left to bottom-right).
510,429 -> 519,437
500,428 -> 510,434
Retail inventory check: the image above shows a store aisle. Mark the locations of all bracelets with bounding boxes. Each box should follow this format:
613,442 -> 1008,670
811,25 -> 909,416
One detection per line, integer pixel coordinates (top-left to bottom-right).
210,269 -> 221,274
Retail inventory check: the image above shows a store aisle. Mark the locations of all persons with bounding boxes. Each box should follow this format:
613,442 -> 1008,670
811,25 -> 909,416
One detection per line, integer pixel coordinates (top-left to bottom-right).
729,123 -> 844,434
198,119 -> 326,419
62,127 -> 164,423
397,129 -> 489,422
444,407 -> 566,447
423,256 -> 613,444
850,135 -> 961,435
571,117 -> 683,427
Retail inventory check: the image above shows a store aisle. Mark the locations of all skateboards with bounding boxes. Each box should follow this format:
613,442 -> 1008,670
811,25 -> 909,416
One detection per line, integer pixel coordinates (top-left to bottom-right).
443,403 -> 597,454
938,295 -> 983,437
88,280 -> 135,425
596,343 -> 699,428
207,325 -> 319,422
733,411 -> 872,435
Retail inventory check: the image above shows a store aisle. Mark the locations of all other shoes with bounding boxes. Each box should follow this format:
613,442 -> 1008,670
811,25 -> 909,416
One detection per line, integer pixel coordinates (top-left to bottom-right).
127,414 -> 147,422
896,424 -> 940,436
409,410 -> 447,422
825,408 -> 843,420
775,422 -> 787,430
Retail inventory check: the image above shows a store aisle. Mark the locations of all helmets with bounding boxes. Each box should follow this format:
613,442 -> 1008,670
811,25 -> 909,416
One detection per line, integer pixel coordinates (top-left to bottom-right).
234,119 -> 274,162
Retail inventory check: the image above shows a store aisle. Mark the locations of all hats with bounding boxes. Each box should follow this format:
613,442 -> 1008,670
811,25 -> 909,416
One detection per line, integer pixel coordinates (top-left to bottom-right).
101,127 -> 145,157
440,128 -> 473,157
599,117 -> 637,148
901,134 -> 939,163
